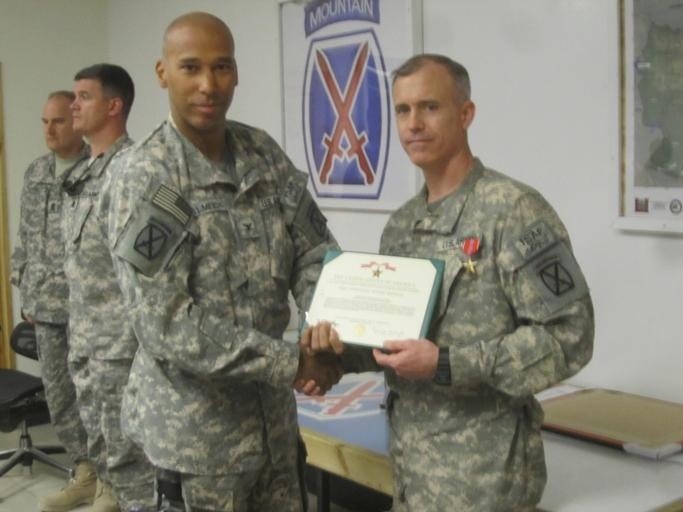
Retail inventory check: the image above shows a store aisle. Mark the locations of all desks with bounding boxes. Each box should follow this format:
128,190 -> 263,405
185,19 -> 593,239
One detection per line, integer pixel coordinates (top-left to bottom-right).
280,329 -> 682,511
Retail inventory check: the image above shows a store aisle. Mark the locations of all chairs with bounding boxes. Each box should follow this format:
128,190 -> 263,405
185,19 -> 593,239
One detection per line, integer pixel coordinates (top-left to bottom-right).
0,306 -> 77,492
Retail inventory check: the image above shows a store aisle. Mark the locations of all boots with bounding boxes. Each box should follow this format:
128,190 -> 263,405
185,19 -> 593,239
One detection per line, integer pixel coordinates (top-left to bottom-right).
92,477 -> 118,512
39,460 -> 97,512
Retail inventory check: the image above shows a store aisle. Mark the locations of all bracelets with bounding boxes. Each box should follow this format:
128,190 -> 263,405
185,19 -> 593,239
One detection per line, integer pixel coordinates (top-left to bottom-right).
432,345 -> 451,386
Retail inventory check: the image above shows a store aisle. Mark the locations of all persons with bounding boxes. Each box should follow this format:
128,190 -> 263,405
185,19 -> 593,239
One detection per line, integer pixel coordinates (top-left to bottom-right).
59,62 -> 169,511
300,50 -> 595,510
6,89 -> 118,512
97,9 -> 346,511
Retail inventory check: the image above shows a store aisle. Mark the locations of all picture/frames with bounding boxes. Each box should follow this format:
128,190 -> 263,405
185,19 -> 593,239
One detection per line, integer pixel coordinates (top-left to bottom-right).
276,1 -> 421,214
617,1 -> 681,239
